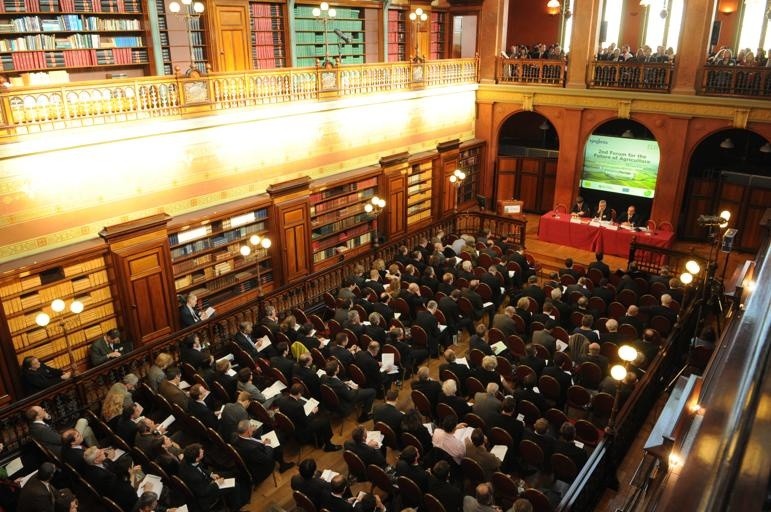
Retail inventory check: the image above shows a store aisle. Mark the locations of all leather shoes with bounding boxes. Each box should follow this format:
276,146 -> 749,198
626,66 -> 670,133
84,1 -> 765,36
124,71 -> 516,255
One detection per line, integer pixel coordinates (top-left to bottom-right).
358,414 -> 374,422
279,461 -> 295,473
324,444 -> 343,452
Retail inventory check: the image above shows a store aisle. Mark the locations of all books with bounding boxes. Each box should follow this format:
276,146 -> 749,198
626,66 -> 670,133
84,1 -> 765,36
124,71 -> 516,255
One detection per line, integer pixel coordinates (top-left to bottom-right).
170,147 -> 483,312
1,1 -> 448,91
570,215 -> 631,231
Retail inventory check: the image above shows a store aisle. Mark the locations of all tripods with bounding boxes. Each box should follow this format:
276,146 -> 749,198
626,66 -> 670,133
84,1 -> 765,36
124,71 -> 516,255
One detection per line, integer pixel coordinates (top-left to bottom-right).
707,253 -> 729,340
680,273 -> 716,376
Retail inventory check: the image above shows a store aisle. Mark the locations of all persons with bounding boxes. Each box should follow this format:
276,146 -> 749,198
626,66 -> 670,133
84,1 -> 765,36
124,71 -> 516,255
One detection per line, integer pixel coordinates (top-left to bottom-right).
571,196 -> 592,218
0,226 -> 729,512
615,205 -> 644,228
591,199 -> 612,221
499,41 -> 771,97
